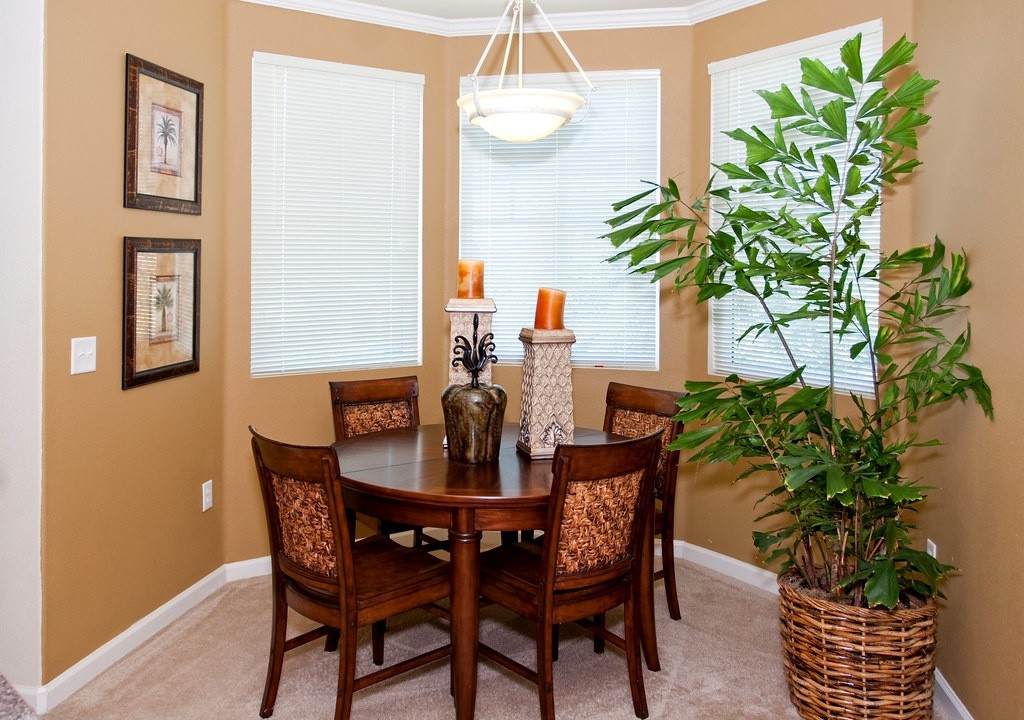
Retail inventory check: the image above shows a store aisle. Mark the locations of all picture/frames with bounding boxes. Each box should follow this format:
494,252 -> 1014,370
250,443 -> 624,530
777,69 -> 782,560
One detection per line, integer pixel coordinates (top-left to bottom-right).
121,236 -> 202,390
124,53 -> 204,215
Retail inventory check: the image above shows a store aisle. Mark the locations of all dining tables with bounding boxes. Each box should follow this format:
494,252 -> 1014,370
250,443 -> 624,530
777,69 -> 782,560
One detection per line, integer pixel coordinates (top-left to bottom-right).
323,423 -> 662,720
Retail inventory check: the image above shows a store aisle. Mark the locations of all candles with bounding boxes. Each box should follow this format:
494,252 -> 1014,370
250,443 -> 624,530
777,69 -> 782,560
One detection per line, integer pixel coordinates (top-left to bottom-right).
534,288 -> 566,330
458,260 -> 485,299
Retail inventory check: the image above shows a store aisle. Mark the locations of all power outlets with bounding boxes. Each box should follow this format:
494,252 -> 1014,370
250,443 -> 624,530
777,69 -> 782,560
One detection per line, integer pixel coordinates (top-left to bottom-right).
202,480 -> 213,512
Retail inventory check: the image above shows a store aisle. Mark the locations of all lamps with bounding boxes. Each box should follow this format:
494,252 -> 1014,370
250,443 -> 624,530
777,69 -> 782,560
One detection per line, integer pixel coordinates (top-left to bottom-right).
457,0 -> 596,143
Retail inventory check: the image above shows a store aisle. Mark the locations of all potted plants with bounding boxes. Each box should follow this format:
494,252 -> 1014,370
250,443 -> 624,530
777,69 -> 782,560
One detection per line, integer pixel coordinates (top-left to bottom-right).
595,31 -> 995,720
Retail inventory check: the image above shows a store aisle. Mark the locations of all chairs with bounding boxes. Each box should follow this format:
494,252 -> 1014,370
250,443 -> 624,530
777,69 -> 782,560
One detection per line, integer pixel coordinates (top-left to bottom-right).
328,376 -> 452,562
478,428 -> 665,720
249,424 -> 455,720
593,382 -> 692,655
